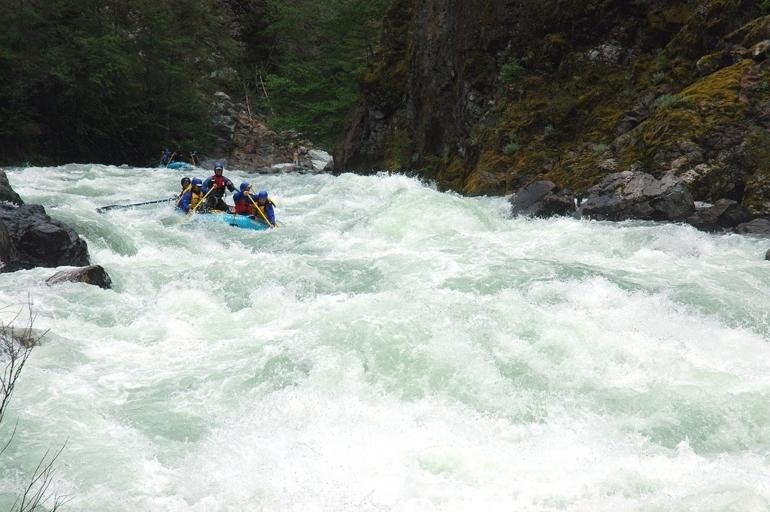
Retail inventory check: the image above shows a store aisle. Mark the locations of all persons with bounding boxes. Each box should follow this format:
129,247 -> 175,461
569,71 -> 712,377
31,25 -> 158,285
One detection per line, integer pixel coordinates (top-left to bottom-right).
159,148 -> 202,165
202,162 -> 238,214
244,189 -> 276,228
233,182 -> 254,216
174,177 -> 189,203
182,177 -> 206,213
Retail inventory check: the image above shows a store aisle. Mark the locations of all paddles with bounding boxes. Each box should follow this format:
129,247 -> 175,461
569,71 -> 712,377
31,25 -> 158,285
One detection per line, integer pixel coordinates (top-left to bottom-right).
97,198 -> 179,215
179,185 -> 216,226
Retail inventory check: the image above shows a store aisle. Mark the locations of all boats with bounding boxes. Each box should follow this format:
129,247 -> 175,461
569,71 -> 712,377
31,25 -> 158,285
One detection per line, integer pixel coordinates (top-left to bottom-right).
170,199 -> 275,233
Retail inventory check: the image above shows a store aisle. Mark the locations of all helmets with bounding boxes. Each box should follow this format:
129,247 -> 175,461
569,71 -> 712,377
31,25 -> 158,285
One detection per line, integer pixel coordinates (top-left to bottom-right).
240,181 -> 251,192
258,191 -> 268,198
181,177 -> 190,186
213,162 -> 224,170
191,178 -> 203,187
